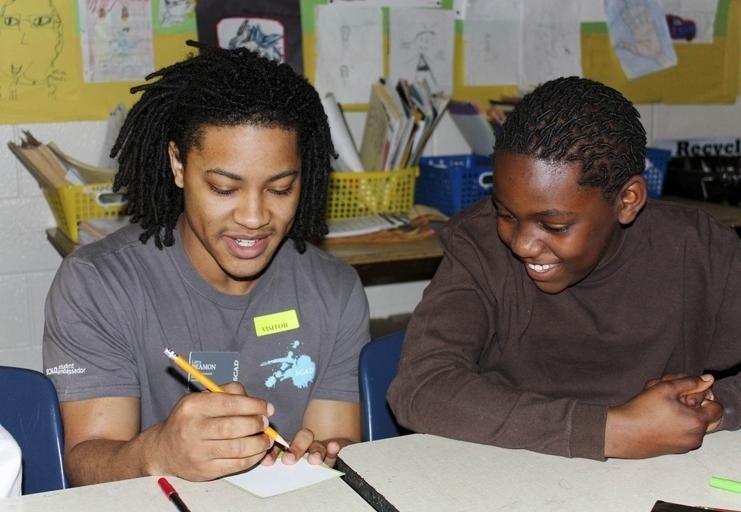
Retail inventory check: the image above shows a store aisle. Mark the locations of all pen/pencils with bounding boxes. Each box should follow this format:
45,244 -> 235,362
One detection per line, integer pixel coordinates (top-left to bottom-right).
158,477 -> 192,512
710,476 -> 741,494
163,347 -> 290,449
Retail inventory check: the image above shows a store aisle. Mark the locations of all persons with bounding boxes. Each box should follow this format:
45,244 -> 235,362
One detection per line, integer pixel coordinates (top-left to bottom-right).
41,35 -> 373,484
384,74 -> 740,465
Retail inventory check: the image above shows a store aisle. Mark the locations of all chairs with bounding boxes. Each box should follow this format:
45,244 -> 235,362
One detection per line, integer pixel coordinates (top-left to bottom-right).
0,366 -> 73,498
358,328 -> 414,443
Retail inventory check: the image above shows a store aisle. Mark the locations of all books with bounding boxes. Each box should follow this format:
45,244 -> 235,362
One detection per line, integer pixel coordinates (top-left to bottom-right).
359,74 -> 449,206
7,126 -> 127,248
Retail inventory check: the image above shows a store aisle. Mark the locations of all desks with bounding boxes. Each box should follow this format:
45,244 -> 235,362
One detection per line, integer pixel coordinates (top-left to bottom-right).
46,207 -> 737,289
0,447 -> 385,511
332,406 -> 741,511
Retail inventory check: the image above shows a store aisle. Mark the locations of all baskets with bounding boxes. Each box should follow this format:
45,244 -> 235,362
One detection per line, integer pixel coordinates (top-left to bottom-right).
416,155 -> 493,220
38,179 -> 133,245
326,166 -> 421,220
643,148 -> 671,198
